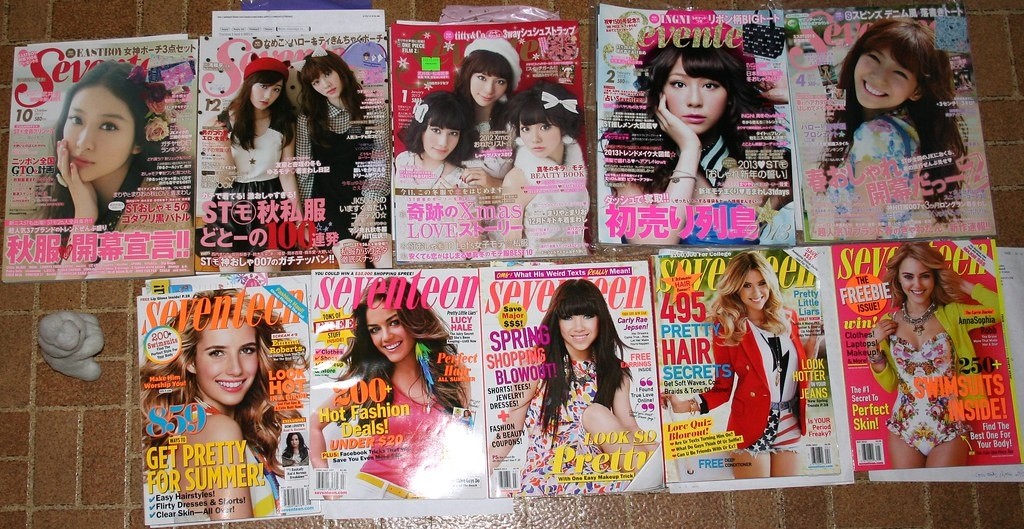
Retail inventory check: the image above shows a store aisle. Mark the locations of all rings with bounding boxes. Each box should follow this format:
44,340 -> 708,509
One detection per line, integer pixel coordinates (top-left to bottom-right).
56,174 -> 67,187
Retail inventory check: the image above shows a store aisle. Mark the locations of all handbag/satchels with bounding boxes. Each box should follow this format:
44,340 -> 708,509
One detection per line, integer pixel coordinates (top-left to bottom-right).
743,10 -> 785,58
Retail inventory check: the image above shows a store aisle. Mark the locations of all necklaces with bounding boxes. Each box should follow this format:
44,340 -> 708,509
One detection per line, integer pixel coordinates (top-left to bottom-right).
750,320 -> 782,387
194,395 -> 280,513
902,302 -> 937,336
702,142 -> 715,153
248,151 -> 256,165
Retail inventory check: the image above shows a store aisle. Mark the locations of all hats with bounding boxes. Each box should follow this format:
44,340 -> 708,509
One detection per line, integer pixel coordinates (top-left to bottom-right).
244,58 -> 289,82
464,35 -> 522,90
635,9 -> 746,68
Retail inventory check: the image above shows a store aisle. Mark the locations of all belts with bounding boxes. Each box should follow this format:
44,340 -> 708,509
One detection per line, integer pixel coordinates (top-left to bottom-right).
771,396 -> 797,410
357,472 -> 418,500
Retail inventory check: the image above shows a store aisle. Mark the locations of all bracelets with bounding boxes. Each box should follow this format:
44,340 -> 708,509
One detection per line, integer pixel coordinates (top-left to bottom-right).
669,176 -> 697,183
688,398 -> 696,416
673,169 -> 692,175
868,350 -> 884,364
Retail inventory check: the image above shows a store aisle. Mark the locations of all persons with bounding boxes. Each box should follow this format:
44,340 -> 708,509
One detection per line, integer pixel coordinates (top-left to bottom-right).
31,9 -> 1002,523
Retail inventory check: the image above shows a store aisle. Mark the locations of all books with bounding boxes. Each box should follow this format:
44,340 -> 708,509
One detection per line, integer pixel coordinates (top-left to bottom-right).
0,0 -> 1024,529
